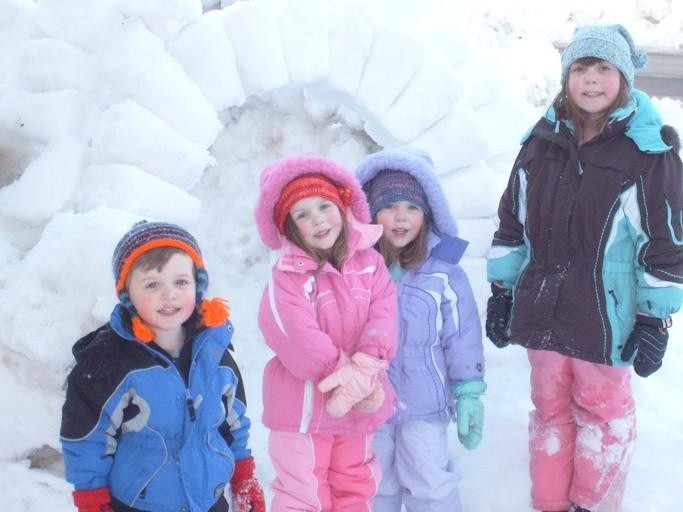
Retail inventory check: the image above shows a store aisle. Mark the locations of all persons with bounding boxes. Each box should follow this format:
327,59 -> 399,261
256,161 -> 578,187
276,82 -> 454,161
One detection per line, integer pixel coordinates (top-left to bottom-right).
352,150 -> 489,511
485,25 -> 681,512
255,157 -> 403,512
58,220 -> 266,511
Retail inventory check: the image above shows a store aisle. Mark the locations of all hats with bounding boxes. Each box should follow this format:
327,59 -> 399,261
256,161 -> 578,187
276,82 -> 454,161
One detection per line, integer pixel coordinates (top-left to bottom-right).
110,222 -> 204,292
559,23 -> 637,93
368,168 -> 428,223
273,172 -> 346,235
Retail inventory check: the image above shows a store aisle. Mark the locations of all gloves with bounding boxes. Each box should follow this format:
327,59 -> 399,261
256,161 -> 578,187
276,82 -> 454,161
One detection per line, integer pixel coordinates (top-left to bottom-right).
71,487 -> 113,511
449,375 -> 487,450
484,282 -> 513,349
329,346 -> 384,414
620,312 -> 673,378
227,455 -> 266,512
316,352 -> 389,419
